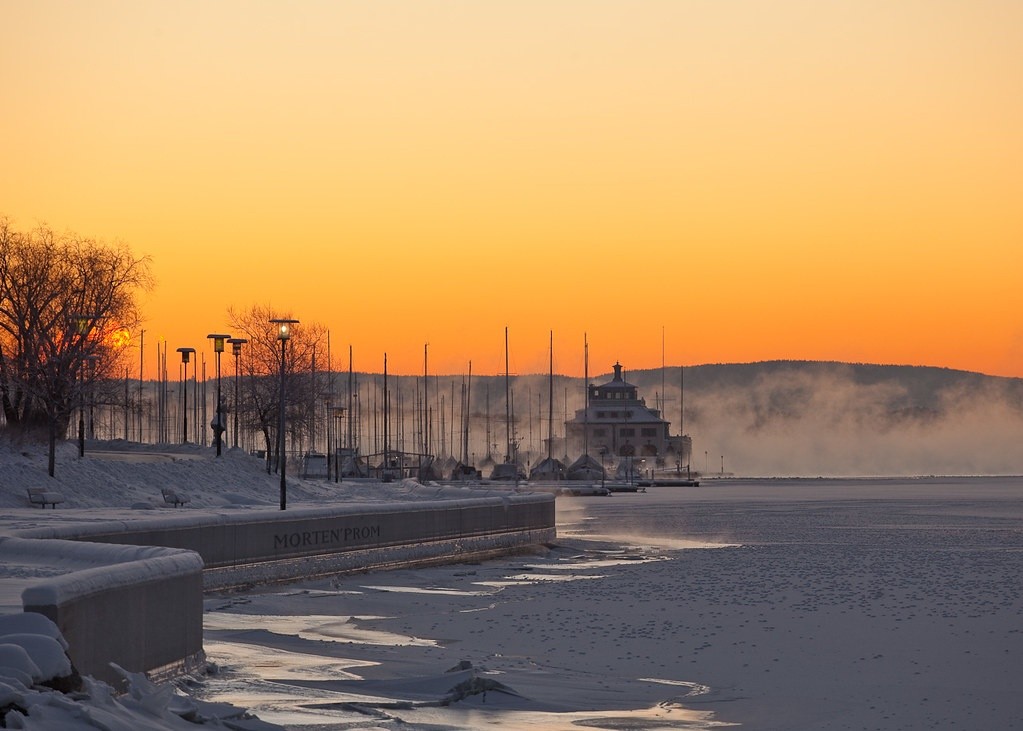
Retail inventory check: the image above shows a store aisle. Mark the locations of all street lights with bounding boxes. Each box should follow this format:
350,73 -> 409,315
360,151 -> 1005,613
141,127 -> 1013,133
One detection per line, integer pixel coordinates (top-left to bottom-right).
176,347 -> 195,441
332,406 -> 347,483
207,334 -> 232,456
226,338 -> 248,447
267,319 -> 299,511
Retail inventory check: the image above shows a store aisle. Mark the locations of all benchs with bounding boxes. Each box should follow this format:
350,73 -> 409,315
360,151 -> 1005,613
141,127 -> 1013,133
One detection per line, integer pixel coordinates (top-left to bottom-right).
26,487 -> 64,509
161,490 -> 191,508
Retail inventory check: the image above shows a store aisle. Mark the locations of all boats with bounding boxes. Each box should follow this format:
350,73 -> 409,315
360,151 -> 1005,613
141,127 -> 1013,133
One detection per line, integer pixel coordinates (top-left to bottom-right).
614,461 -> 642,479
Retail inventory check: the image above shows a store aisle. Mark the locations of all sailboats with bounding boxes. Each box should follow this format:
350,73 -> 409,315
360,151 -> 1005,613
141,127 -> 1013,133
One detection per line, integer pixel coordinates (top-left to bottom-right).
567,332 -> 607,481
530,330 -> 567,480
353,327 -> 528,481
